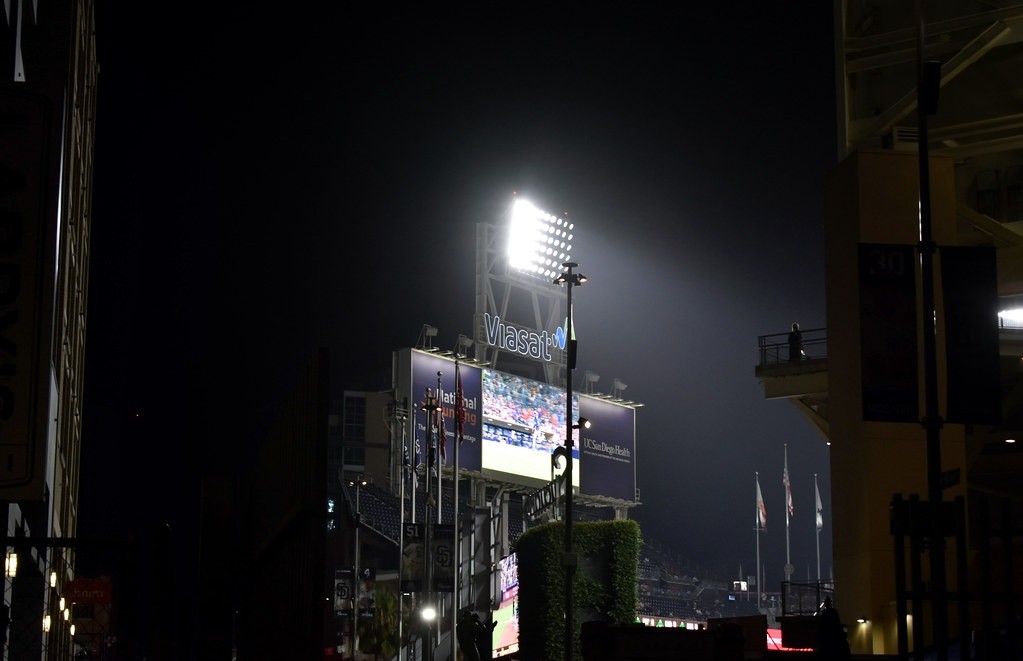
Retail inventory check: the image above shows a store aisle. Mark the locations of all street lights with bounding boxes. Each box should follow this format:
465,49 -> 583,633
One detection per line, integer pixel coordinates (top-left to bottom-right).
555,262 -> 591,661
420,396 -> 442,661
397,592 -> 410,661
347,473 -> 369,661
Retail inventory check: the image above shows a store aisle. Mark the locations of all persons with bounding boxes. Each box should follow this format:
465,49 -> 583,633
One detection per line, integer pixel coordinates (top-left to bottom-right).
482,374 -> 578,450
788,323 -> 802,363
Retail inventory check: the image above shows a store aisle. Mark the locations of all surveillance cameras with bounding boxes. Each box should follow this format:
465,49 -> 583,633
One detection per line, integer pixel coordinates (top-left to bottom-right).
425,496 -> 436,509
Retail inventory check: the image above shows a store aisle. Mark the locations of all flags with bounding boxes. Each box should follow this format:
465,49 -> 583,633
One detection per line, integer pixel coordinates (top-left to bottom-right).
457,368 -> 464,443
757,481 -> 766,529
415,427 -> 420,480
783,466 -> 793,515
430,418 -> 437,468
816,484 -> 823,529
438,395 -> 446,462
404,436 -> 410,483
